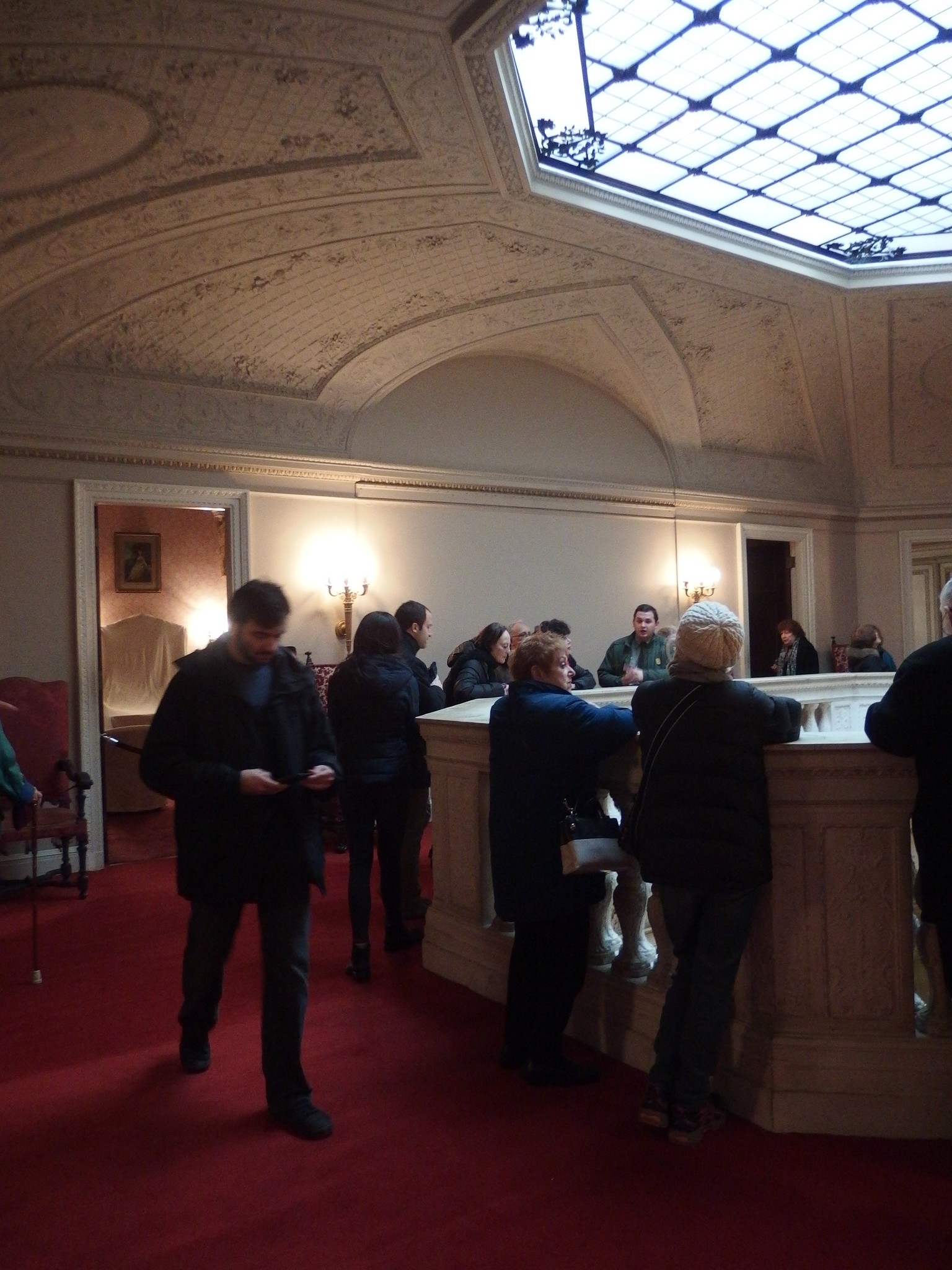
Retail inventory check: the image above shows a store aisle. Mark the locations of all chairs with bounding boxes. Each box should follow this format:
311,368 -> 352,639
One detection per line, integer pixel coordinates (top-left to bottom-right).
0,676 -> 94,900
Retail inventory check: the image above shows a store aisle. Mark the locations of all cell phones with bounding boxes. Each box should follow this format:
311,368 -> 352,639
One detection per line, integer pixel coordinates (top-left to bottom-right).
275,773 -> 313,784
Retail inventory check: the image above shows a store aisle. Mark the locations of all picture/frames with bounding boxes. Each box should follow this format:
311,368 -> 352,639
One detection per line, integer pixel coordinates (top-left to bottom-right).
113,532 -> 163,594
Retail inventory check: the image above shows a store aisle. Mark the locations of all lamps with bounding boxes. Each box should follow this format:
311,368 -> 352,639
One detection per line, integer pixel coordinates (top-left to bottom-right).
681,548 -> 720,605
319,536 -> 379,653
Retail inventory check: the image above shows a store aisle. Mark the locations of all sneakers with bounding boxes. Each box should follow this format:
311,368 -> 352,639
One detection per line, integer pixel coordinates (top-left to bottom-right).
383,922 -> 420,952
497,1033 -> 533,1066
669,1101 -> 725,1146
346,942 -> 372,982
640,1083 -> 672,1129
179,1023 -> 211,1072
265,1091 -> 333,1137
526,1053 -> 600,1086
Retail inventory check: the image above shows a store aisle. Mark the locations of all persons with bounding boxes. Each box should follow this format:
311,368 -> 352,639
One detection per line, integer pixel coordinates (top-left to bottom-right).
484,626 -> 639,1085
441,603 -> 896,706
144,578 -> 341,1135
393,600 -> 446,924
631,600 -> 801,1147
864,576 -> 952,1027
1,719 -> 47,807
332,610 -> 429,986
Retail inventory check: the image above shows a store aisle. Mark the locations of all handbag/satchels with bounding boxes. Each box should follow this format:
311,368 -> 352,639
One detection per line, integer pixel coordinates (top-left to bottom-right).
616,754 -> 655,851
547,799 -> 625,877
